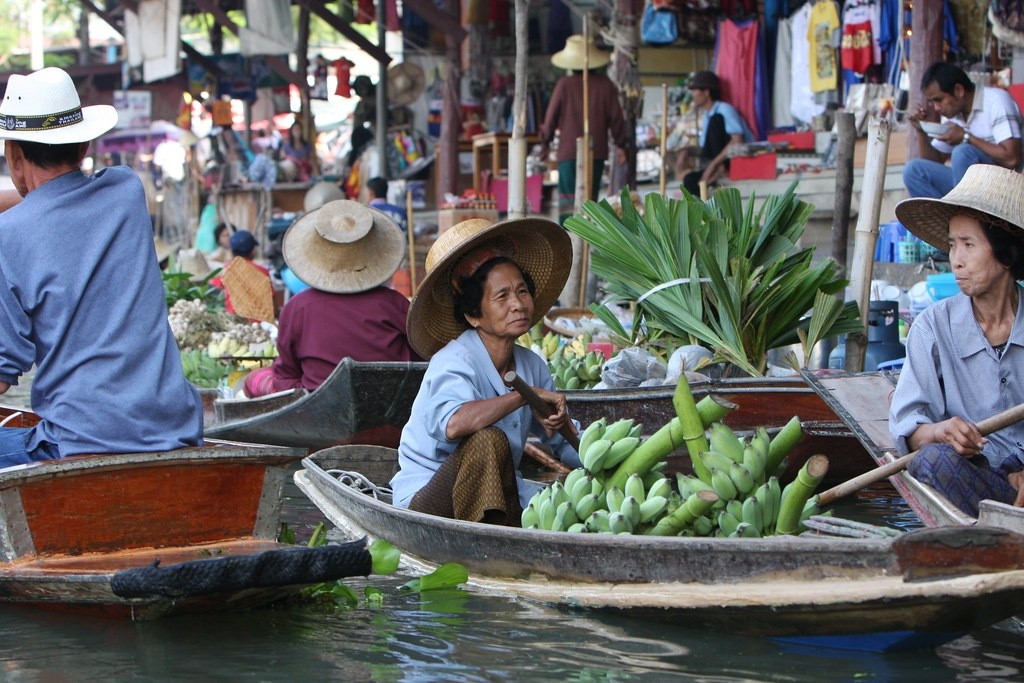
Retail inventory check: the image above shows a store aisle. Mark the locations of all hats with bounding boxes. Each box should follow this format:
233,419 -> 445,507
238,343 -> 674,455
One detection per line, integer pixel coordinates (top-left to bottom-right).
684,69 -> 721,89
304,183 -> 347,212
895,165 -> 1024,279
229,229 -> 260,257
405,218 -> 576,361
348,76 -> 377,90
551,35 -> 611,70
384,62 -> 427,105
281,198 -> 408,294
0,67 -> 119,145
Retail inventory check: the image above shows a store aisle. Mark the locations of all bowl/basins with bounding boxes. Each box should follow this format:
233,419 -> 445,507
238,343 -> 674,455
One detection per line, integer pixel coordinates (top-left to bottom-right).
870,279 -> 934,311
919,120 -> 951,137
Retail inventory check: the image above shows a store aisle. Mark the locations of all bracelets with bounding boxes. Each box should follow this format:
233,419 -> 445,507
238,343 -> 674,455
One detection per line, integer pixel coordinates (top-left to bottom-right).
962,131 -> 972,145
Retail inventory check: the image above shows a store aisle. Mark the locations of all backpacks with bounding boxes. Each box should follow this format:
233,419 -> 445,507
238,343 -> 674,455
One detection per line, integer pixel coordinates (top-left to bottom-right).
640,3 -> 678,43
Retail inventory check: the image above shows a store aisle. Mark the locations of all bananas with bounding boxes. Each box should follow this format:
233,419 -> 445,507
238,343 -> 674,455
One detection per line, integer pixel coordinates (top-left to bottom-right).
182,333 -> 279,387
521,418 -> 833,534
517,331 -> 607,390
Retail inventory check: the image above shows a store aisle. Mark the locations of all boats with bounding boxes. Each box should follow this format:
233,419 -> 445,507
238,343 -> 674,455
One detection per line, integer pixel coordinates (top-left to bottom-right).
187,357 -> 431,505
293,444 -> 1024,653
799,364 -> 1024,541
0,400 -> 372,622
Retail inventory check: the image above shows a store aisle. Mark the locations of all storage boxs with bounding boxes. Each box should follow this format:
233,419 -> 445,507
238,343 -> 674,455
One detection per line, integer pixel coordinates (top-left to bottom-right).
728,129 -> 814,180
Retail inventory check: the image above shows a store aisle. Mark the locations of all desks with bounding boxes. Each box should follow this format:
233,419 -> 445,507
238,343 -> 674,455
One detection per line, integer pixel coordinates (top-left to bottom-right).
433,131 -> 556,199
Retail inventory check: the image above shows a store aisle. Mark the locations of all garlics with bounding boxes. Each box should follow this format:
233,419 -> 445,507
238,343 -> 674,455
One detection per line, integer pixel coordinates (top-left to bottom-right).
167,298 -> 270,344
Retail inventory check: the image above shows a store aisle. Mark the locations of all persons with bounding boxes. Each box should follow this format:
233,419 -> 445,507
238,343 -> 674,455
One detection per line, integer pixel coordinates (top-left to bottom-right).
243,199 -> 424,402
341,75 -> 379,182
942,39 -> 957,64
902,60 -> 1024,200
205,221 -> 278,323
487,71 -> 535,136
387,247 -> 585,527
250,117 -> 325,180
682,71 -> 758,196
887,163 -> 1024,521
538,44 -> 632,232
0,67 -> 205,467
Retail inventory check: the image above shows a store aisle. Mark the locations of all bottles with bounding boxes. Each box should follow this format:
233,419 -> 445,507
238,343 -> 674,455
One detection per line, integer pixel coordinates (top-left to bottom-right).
898,319 -> 907,338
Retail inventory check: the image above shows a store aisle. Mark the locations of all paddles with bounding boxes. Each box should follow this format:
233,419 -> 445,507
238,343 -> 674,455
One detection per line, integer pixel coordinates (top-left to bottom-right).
505,367 -> 582,454
812,401 -> 1024,513
